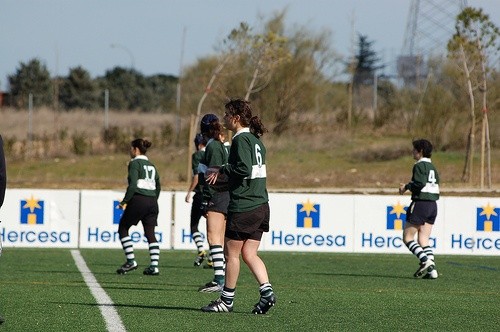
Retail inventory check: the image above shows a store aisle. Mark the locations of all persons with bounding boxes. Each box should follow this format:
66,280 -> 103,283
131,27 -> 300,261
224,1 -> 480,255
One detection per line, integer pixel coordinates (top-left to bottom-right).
218,125 -> 232,152
198,114 -> 230,293
113,138 -> 161,274
185,133 -> 215,269
198,97 -> 276,314
399,139 -> 441,279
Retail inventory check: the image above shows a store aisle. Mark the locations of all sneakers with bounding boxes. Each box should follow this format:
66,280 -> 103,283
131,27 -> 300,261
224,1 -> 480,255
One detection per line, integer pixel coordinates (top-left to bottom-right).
204,259 -> 214,269
252,289 -> 276,314
143,266 -> 160,276
201,297 -> 234,312
414,257 -> 436,277
117,260 -> 138,274
194,251 -> 207,267
198,281 -> 228,293
423,269 -> 438,279
